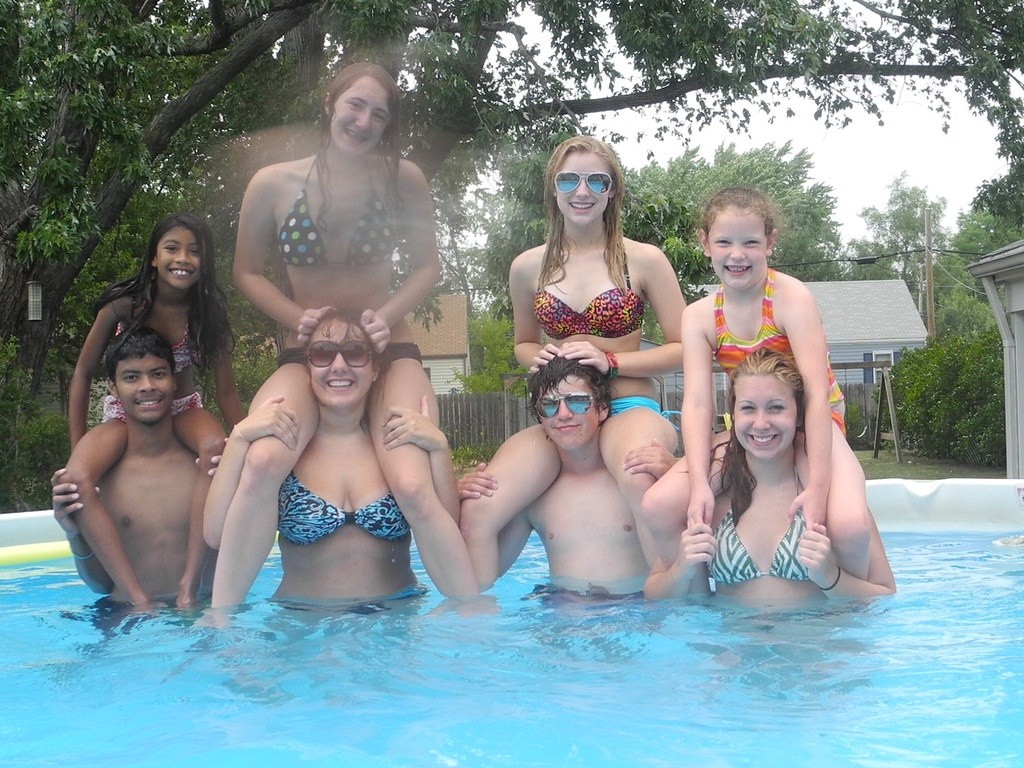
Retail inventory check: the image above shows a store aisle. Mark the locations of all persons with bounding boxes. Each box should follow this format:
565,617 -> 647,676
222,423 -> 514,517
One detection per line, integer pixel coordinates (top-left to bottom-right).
641,347 -> 897,599
209,64 -> 478,607
55,213 -> 248,605
458,352 -> 678,580
456,136 -> 688,580
50,326 -> 226,601
640,187 -> 870,585
203,306 -> 462,602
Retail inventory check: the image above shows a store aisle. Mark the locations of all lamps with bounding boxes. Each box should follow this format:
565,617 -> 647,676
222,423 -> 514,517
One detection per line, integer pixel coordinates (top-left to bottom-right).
26,262 -> 43,322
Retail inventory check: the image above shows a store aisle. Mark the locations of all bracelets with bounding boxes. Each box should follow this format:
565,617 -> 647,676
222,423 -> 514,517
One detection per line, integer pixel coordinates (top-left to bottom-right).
818,566 -> 841,591
603,351 -> 619,381
233,425 -> 252,445
71,552 -> 94,560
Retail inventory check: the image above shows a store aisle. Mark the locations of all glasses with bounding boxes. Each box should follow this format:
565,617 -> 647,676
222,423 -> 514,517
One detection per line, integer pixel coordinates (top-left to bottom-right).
534,392 -> 593,419
305,341 -> 373,367
554,171 -> 613,195
